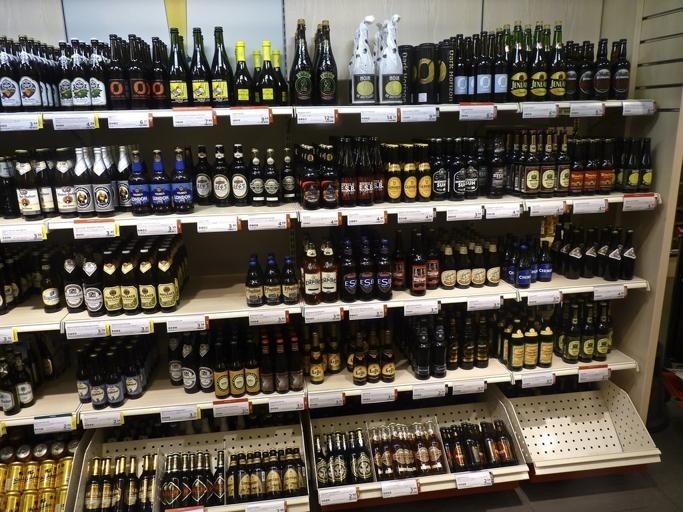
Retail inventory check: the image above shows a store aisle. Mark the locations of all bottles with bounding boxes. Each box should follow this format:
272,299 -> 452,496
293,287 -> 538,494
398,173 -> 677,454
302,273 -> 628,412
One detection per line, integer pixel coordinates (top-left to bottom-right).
0,215 -> 638,318
314,417 -> 519,485
0,123 -> 645,223
80,443 -> 306,511
1,294 -> 618,415
0,13 -> 634,116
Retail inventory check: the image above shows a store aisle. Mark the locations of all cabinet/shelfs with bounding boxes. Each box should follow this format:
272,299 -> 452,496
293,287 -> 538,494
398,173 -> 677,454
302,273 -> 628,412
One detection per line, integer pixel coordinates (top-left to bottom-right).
0,95 -> 667,510
